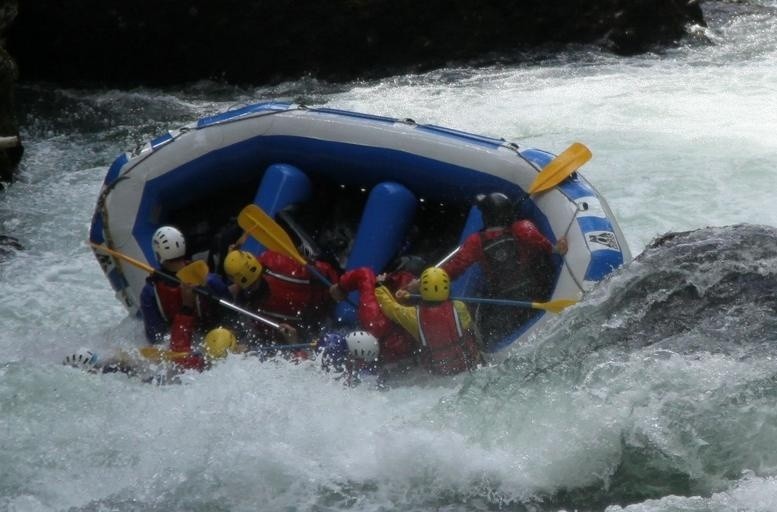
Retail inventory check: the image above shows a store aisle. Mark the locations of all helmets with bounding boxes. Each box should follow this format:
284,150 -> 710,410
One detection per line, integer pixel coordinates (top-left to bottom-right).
480,192 -> 513,227
151,226 -> 186,260
419,267 -> 450,302
63,346 -> 97,372
344,331 -> 380,363
223,250 -> 262,289
203,328 -> 235,359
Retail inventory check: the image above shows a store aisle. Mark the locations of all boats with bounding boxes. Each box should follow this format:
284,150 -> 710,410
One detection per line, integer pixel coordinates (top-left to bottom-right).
90,96 -> 636,395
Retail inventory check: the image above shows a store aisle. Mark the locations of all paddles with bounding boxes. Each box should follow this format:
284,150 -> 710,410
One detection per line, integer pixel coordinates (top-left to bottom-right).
402,294 -> 580,314
435,143 -> 592,269
237,204 -> 360,312
136,344 -> 313,361
85,241 -> 282,330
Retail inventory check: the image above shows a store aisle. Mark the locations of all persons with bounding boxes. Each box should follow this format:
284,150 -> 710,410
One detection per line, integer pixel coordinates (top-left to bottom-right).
99,223 -> 488,386
407,192 -> 568,327
